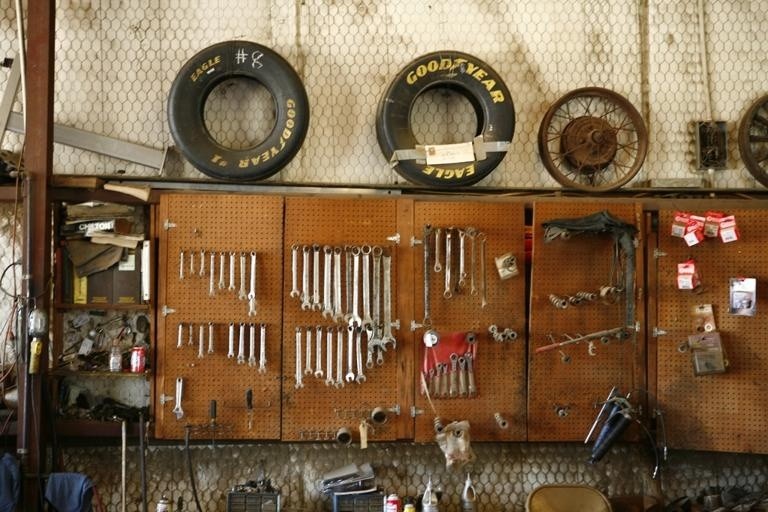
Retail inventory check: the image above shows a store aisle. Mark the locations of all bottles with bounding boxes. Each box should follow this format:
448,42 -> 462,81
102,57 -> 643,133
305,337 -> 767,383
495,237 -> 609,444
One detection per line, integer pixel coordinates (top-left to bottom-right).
109,339 -> 122,372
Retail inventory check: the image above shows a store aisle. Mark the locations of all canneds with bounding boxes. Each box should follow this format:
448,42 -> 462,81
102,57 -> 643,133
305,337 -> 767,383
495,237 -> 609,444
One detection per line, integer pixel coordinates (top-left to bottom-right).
131,347 -> 146,373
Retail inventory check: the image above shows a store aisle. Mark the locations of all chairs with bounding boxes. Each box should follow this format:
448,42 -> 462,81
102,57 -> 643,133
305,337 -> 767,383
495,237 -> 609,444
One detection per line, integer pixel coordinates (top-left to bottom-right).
525,485 -> 613,512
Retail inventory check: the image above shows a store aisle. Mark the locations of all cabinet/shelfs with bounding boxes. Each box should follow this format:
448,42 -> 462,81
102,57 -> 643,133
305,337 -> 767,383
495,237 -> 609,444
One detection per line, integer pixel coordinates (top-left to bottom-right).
50,178 -> 155,445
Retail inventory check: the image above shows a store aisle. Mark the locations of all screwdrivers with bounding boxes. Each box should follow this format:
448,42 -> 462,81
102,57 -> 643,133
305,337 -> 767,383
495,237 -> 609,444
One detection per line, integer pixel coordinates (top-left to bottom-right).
209,400 -> 218,453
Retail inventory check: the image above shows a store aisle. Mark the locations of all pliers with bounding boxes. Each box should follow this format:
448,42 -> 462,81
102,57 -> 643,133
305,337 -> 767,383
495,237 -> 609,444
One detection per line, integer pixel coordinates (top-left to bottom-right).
172,377 -> 184,421
